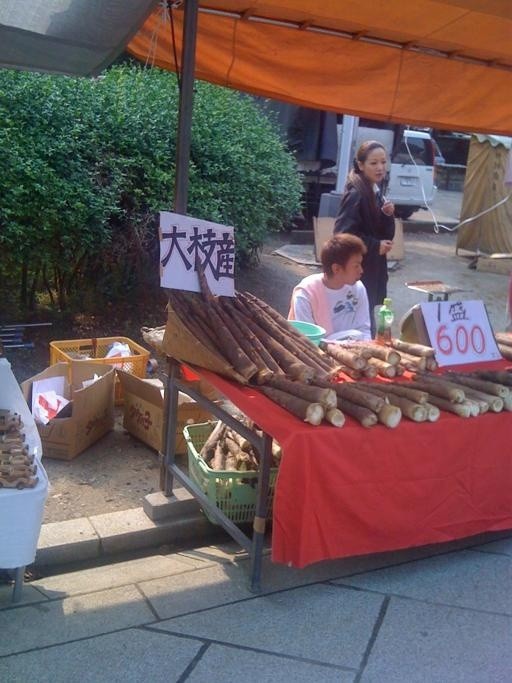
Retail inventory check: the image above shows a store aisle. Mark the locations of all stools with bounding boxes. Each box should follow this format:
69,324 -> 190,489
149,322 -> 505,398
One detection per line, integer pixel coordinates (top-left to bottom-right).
402,279 -> 469,305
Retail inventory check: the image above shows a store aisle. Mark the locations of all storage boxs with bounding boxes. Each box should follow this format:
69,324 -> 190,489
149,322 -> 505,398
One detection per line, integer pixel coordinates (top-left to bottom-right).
48,335 -> 152,409
182,419 -> 283,528
456,246 -> 512,277
18,360 -> 117,463
116,368 -> 214,458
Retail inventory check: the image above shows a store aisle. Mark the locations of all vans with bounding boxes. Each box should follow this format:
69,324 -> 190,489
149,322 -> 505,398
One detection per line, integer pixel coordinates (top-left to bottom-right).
333,124 -> 435,219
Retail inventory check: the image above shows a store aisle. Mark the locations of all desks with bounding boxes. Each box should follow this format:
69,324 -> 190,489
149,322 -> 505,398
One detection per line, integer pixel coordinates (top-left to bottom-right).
158,351 -> 512,595
1,357 -> 49,605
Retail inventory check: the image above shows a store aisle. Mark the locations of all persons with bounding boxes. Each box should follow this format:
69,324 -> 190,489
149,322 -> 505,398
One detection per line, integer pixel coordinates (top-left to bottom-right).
334,141 -> 395,340
287,233 -> 373,344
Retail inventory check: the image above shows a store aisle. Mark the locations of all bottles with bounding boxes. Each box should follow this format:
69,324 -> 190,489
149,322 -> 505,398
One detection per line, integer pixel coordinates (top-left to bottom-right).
375,297 -> 394,347
